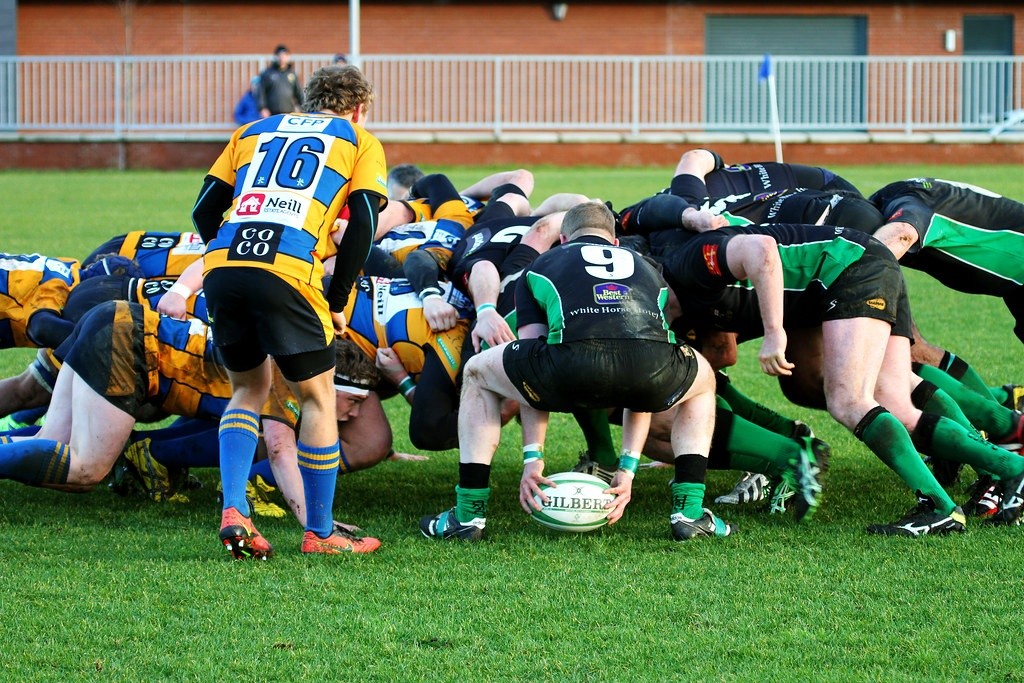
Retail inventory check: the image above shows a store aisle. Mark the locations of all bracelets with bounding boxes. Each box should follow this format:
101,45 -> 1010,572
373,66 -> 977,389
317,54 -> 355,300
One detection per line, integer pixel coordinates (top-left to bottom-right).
523,443 -> 544,465
167,281 -> 194,300
398,375 -> 417,397
475,303 -> 496,316
617,448 -> 641,480
418,287 -> 441,303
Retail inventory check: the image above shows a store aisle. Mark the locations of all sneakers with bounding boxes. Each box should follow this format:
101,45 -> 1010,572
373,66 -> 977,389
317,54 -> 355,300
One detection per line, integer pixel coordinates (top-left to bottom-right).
1001,384 -> 1024,414
748,477 -> 795,515
961,474 -> 1002,517
671,508 -> 730,539
220,507 -> 273,557
419,507 -> 486,542
791,437 -> 832,523
302,529 -> 381,554
866,490 -> 965,537
120,437 -> 190,503
571,450 -> 619,485
715,472 -> 771,504
982,471 -> 1024,528
991,410 -> 1024,457
216,474 -> 288,520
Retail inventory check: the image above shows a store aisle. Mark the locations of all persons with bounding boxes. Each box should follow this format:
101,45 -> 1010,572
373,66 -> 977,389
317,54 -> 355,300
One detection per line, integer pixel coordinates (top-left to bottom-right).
188,64 -> 389,557
0,148 -> 1024,540
333,53 -> 347,65
234,43 -> 303,126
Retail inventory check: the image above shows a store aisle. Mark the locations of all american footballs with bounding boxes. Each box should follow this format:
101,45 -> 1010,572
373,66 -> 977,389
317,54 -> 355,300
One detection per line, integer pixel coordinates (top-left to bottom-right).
526,472 -> 616,532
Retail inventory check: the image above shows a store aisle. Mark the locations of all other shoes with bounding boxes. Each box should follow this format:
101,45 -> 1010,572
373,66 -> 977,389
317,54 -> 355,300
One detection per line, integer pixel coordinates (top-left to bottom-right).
1,415 -> 44,431
